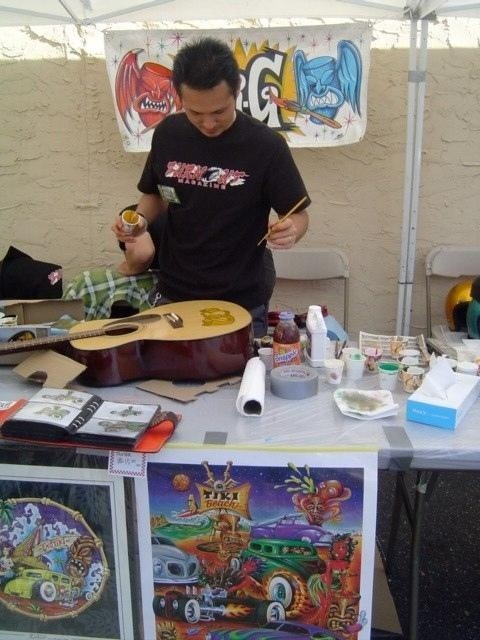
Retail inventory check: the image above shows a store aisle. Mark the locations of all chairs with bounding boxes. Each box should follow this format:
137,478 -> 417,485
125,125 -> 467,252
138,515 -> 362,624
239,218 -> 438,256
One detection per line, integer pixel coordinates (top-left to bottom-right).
268,247 -> 349,340
423,243 -> 480,339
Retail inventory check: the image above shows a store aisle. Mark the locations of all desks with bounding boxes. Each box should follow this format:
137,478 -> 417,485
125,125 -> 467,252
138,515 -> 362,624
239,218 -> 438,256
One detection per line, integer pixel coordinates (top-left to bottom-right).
1,339 -> 479,640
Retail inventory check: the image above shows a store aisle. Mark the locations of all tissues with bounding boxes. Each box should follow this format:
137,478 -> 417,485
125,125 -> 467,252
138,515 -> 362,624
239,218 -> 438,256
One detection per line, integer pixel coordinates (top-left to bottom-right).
406,354 -> 480,430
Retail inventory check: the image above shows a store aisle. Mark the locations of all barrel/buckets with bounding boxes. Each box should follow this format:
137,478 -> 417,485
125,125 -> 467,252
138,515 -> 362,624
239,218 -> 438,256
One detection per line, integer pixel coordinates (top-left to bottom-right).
304,305 -> 327,368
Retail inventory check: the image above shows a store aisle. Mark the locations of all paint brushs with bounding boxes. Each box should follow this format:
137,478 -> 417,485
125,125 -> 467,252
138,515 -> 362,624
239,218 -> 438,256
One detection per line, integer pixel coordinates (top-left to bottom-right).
256,195 -> 308,247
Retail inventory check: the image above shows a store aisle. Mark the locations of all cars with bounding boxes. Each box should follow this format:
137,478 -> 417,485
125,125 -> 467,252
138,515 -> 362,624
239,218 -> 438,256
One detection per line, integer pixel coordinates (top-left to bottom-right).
150,533 -> 324,627
3,570 -> 78,604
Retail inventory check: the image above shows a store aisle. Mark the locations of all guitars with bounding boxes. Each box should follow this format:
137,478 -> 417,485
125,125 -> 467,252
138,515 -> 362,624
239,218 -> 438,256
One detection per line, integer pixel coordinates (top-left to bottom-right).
1,300 -> 254,387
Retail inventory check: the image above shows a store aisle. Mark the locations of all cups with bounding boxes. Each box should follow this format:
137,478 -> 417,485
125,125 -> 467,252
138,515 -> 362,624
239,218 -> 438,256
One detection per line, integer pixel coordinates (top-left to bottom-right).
257,347 -> 274,371
121,209 -> 141,232
326,342 -> 478,394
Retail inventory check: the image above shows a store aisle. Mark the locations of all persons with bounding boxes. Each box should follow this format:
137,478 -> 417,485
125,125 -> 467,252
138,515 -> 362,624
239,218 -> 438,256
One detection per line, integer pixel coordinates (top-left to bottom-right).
112,36 -> 311,337
117,204 -> 159,276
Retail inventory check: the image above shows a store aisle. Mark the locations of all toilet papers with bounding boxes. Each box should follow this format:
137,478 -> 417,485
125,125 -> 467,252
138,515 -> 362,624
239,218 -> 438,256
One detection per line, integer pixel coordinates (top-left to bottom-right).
235,356 -> 266,417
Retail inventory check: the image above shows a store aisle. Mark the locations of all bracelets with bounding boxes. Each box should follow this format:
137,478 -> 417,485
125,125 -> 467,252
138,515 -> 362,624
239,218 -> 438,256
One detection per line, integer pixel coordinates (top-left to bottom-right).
137,212 -> 144,217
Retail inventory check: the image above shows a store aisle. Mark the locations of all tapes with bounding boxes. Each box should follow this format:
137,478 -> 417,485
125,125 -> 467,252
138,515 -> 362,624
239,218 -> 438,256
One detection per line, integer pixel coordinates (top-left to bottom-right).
269,363 -> 319,401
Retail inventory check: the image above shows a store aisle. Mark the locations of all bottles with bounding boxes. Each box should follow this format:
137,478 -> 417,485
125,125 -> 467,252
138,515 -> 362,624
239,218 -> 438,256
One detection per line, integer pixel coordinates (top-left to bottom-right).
273,310 -> 301,366
